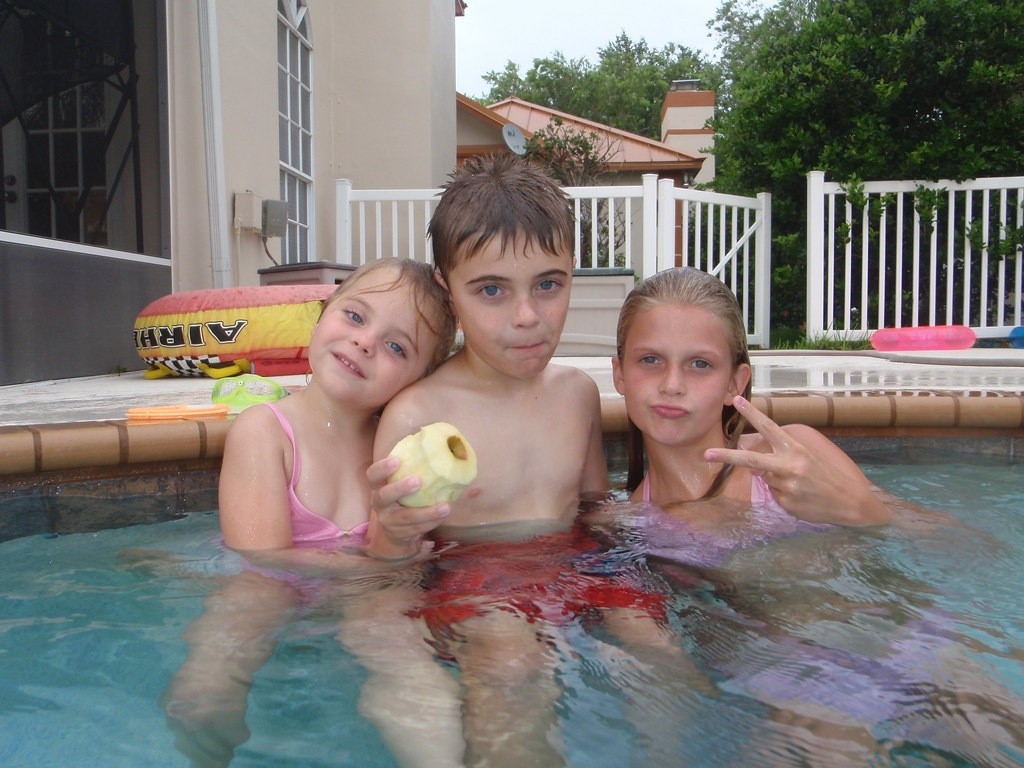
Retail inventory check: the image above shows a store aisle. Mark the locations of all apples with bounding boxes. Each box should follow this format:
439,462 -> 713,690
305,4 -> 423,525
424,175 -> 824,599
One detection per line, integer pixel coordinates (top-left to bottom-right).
382,422 -> 477,509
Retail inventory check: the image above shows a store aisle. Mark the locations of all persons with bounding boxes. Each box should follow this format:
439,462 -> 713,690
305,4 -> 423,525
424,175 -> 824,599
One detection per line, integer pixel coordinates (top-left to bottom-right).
611,266 -> 894,530
217,255 -> 456,553
362,150 -> 608,560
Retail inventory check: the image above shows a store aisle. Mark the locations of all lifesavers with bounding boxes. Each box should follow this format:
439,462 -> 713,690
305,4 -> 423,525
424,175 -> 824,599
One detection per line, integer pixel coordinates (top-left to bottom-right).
129,285 -> 340,376
870,326 -> 977,351
1008,326 -> 1024,350
